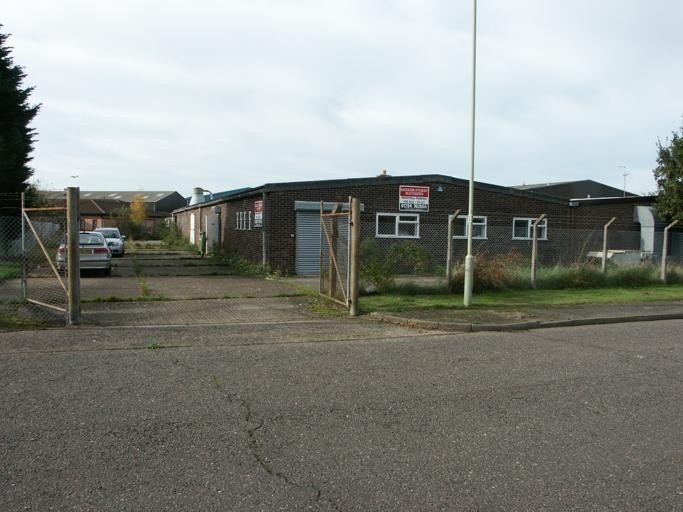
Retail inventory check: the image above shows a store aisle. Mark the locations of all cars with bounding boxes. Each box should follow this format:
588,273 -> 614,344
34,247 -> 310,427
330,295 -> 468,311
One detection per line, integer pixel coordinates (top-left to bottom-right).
92,227 -> 127,257
56,231 -> 112,276
